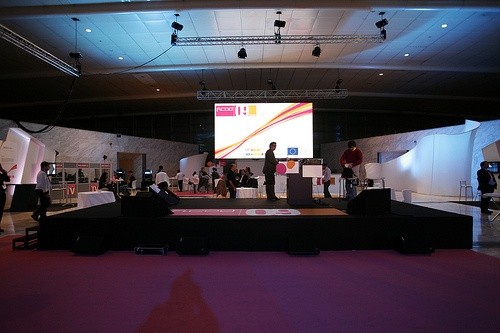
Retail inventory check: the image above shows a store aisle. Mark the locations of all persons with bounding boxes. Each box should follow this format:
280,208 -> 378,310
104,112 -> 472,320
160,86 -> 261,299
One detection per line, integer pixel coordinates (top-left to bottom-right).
263,142 -> 280,202
75,163 -> 254,198
340,141 -> 362,200
476,161 -> 497,215
31,161 -> 51,222
322,164 -> 331,199
0,163 -> 11,233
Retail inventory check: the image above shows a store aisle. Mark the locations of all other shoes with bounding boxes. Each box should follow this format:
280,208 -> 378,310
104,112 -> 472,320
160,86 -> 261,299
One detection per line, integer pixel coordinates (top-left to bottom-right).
487,210 -> 493,213
481,211 -> 491,215
267,198 -> 276,201
0,228 -> 4,232
31,215 -> 39,222
274,196 -> 280,200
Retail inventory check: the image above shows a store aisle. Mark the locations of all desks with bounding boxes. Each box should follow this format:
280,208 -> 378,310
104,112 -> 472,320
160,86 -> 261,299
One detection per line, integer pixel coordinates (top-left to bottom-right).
59,181 -> 75,206
77,191 -> 116,209
168,178 -> 177,192
235,187 -> 259,199
111,180 -> 125,196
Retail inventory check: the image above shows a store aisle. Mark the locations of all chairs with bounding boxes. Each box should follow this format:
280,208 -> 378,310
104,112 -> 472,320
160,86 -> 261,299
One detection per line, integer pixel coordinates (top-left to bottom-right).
126,180 -> 137,196
96,181 -> 109,191
482,193 -> 500,222
460,181 -> 473,197
63,184 -> 78,206
178,178 -> 190,191
351,163 -> 386,190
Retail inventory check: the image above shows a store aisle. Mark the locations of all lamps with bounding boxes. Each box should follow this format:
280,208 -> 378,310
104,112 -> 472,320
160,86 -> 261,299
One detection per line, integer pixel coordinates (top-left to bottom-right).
69,52 -> 84,75
167,11 -> 392,96
